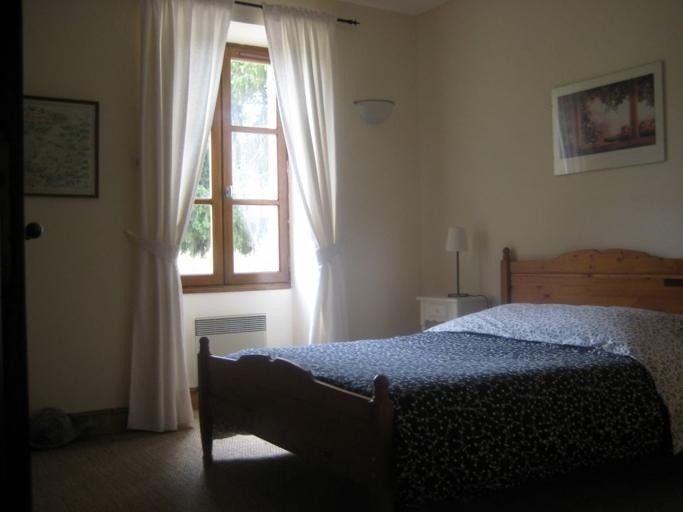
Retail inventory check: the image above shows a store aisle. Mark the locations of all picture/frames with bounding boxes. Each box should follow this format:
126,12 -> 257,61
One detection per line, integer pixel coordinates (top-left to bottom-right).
551,60 -> 666,175
23,94 -> 99,198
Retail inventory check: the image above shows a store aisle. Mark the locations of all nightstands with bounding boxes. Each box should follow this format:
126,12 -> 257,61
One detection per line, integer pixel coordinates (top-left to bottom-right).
416,294 -> 487,331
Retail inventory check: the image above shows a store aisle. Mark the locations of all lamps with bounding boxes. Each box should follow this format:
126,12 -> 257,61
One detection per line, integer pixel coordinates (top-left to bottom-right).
445,226 -> 467,297
354,98 -> 395,124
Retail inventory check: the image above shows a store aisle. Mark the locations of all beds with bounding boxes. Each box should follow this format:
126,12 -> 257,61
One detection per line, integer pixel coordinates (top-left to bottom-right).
197,248 -> 683,512
